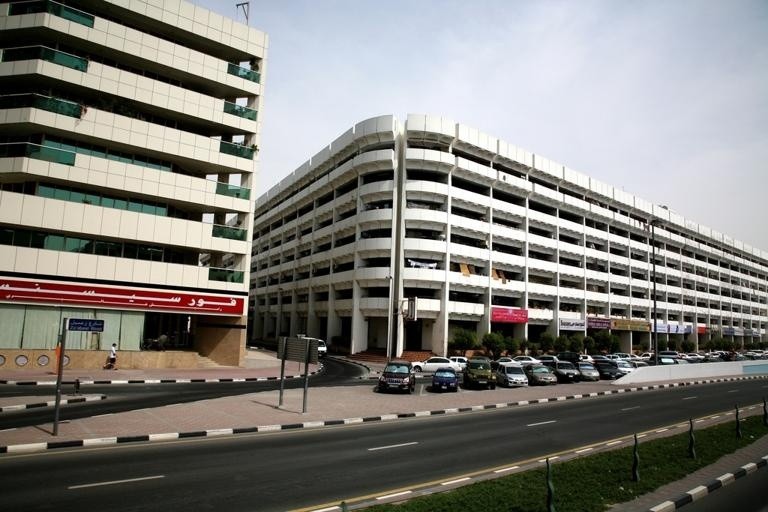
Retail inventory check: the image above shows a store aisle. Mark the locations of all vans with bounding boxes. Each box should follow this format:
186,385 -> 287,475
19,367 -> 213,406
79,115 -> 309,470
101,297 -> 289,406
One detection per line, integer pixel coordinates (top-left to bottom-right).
301,336 -> 327,357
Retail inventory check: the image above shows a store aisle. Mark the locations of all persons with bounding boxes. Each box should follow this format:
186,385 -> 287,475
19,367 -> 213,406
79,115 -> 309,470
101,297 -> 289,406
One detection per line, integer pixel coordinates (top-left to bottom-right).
103,343 -> 119,370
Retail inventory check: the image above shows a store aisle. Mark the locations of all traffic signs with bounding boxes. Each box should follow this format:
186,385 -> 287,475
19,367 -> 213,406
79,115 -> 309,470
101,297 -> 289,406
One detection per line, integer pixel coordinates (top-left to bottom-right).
69,319 -> 104,331
